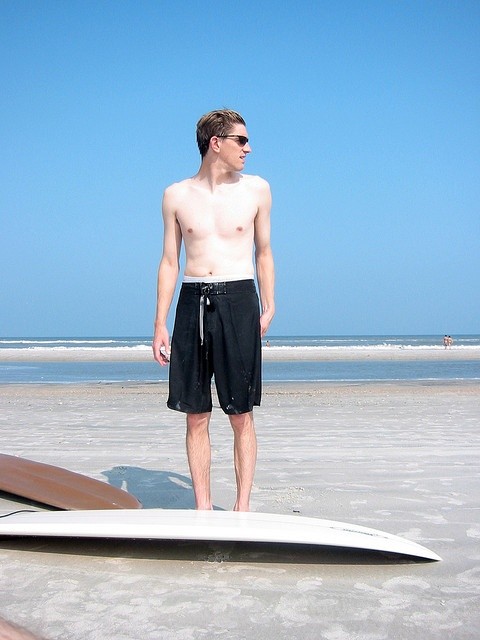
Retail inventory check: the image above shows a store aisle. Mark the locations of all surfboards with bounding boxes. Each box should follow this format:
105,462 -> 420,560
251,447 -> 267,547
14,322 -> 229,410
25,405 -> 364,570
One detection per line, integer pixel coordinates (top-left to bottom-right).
1,453 -> 141,510
1,509 -> 442,563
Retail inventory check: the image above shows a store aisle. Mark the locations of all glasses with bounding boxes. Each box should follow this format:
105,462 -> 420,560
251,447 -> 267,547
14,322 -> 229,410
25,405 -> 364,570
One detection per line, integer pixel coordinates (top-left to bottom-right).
217,135 -> 249,145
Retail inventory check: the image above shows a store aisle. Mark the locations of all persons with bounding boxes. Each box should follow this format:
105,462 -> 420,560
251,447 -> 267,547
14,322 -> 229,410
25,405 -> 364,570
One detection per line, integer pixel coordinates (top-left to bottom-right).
442,334 -> 454,349
151,108 -> 276,510
